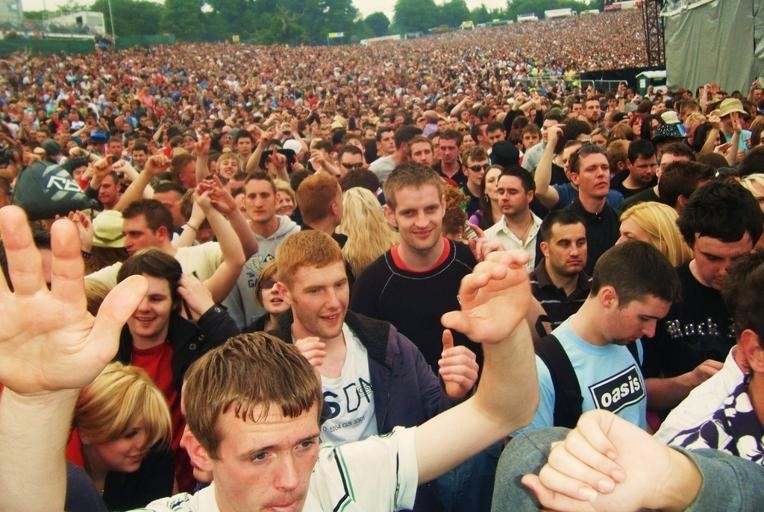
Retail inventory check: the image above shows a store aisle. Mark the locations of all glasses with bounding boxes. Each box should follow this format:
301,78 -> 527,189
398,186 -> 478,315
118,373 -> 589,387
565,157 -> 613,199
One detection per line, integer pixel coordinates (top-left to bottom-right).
466,163 -> 488,171
341,162 -> 362,168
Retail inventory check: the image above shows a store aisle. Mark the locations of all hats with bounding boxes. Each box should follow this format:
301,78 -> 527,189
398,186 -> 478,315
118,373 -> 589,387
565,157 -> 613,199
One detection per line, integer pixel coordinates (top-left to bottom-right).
661,111 -> 680,124
11,161 -> 92,220
652,125 -> 687,142
92,209 -> 125,248
718,98 -> 750,118
43,142 -> 60,156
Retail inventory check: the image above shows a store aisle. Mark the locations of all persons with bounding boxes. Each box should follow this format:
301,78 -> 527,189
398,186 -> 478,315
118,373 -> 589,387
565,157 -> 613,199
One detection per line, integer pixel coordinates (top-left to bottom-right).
0,201 -> 541,511
2,6 -> 764,459
487,406 -> 764,512
71,361 -> 173,510
